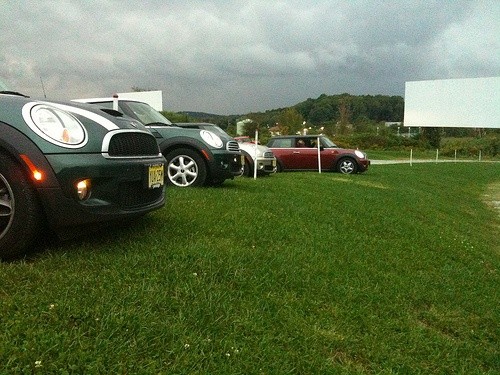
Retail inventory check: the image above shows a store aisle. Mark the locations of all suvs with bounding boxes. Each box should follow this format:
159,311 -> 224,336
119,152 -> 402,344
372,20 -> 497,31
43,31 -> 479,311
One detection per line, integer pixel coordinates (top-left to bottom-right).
0,88 -> 168,261
68,96 -> 243,192
265,135 -> 371,174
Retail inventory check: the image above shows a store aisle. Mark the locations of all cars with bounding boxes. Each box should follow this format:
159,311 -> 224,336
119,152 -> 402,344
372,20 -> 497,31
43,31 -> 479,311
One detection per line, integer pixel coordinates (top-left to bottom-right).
230,141 -> 277,179
